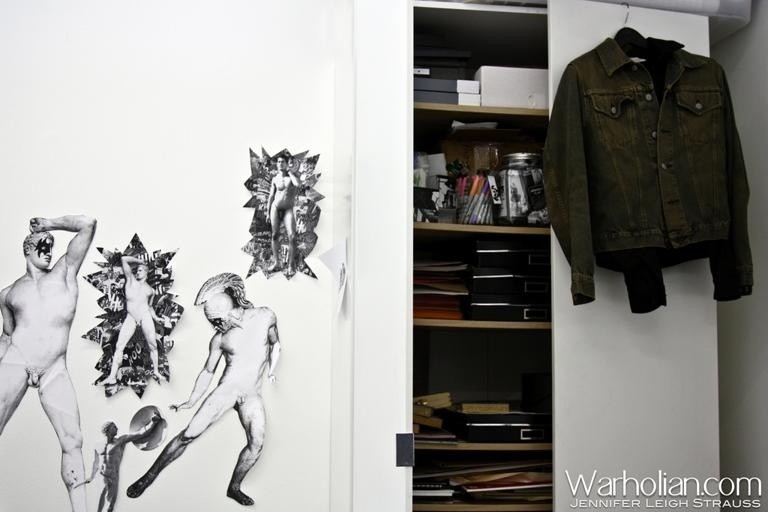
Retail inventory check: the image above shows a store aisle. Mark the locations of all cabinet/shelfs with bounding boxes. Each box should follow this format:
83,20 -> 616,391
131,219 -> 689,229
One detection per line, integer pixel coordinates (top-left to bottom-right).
2,0 -> 720,512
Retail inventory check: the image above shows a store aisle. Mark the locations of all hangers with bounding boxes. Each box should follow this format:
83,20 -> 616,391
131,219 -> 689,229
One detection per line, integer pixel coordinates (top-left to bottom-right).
613,2 -> 651,49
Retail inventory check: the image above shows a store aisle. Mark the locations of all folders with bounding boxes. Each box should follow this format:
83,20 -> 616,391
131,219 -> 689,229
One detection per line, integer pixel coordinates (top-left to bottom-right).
414,67 -> 481,107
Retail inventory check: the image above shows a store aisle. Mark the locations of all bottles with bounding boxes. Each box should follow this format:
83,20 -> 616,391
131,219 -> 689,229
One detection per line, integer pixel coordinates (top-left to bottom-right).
496,152 -> 550,227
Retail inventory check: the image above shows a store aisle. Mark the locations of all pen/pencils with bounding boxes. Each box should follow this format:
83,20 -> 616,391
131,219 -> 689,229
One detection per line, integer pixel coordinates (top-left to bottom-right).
456,175 -> 494,225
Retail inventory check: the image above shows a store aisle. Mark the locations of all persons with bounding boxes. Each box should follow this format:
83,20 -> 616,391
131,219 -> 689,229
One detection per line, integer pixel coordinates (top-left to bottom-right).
0,151 -> 312,512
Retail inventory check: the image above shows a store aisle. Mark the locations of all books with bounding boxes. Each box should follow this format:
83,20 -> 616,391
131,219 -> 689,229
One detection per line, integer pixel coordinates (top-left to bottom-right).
412,254 -> 471,322
412,387 -> 554,508
